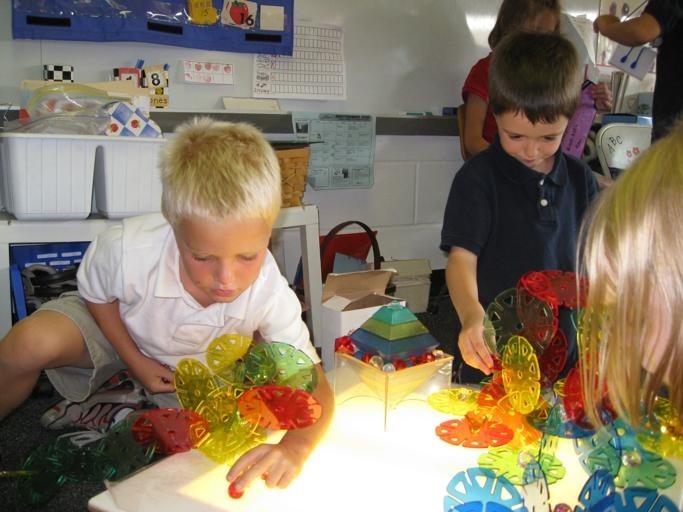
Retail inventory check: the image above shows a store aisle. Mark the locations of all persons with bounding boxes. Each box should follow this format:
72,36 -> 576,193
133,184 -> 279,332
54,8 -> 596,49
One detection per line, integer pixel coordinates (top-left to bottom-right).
461,1 -> 613,157
572,119 -> 682,441
436,30 -> 600,387
593,1 -> 681,145
0,115 -> 337,498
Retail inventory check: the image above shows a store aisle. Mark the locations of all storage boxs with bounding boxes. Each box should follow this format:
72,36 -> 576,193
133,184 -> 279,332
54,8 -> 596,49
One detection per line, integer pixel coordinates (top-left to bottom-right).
321,260 -> 432,371
1,132 -> 168,220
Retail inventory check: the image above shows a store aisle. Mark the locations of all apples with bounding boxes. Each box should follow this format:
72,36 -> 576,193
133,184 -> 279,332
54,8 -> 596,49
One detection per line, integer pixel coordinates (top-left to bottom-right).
230,1 -> 248,24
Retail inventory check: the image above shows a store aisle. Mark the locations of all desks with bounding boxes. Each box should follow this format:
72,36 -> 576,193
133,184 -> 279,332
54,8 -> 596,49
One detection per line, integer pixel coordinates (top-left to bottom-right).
0,205 -> 324,348
87,366 -> 683,512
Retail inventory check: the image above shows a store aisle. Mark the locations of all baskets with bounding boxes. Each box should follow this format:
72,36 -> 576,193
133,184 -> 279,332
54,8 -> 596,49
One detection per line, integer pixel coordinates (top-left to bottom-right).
273,147 -> 309,208
